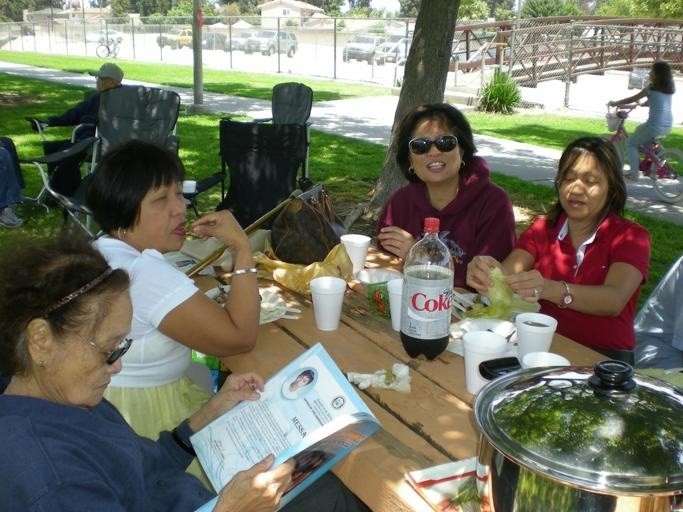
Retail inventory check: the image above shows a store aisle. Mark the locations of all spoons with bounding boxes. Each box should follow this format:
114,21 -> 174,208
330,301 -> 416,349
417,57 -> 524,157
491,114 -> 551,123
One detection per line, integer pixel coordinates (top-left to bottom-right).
262,298 -> 301,315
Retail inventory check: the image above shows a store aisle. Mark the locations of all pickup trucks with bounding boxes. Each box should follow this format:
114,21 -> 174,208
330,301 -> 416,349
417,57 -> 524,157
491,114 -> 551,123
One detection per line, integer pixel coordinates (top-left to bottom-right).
245,29 -> 297,58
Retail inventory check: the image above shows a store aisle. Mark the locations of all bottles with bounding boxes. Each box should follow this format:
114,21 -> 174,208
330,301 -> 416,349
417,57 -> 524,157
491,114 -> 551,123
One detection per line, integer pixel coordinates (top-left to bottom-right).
400,217 -> 454,362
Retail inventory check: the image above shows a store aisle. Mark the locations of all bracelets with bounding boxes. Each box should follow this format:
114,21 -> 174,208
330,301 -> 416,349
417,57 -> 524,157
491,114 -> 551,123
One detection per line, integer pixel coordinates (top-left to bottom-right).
234,266 -> 258,279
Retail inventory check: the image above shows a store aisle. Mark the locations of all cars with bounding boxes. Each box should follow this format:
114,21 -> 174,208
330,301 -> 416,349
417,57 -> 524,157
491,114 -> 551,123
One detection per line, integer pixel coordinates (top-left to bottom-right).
87,30 -> 125,43
191,32 -> 250,51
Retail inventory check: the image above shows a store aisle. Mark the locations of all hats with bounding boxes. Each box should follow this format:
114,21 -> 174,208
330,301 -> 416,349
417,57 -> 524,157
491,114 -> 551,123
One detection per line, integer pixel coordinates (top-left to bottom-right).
88,63 -> 123,82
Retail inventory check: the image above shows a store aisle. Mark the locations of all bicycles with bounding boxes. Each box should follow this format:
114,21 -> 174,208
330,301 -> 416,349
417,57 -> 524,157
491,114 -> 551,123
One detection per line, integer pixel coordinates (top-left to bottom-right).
599,100 -> 681,204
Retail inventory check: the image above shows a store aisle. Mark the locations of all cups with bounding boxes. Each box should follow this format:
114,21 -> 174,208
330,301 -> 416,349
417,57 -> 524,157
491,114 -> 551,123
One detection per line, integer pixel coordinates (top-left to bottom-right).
340,234 -> 372,278
515,311 -> 558,358
386,278 -> 403,332
309,276 -> 346,332
522,350 -> 572,371
462,330 -> 509,396
182,180 -> 196,193
355,267 -> 402,298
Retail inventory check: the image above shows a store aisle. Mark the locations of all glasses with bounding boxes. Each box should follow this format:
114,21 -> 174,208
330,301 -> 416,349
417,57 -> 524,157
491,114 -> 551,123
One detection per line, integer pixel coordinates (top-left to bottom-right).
408,135 -> 458,156
76,332 -> 132,364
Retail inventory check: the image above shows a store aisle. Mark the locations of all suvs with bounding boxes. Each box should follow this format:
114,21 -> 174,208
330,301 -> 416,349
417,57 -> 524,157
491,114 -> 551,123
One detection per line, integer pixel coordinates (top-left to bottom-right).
157,29 -> 192,50
342,35 -> 407,67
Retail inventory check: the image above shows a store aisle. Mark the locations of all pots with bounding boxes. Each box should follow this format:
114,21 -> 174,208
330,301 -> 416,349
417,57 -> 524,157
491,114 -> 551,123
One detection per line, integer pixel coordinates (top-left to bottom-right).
472,359 -> 683,512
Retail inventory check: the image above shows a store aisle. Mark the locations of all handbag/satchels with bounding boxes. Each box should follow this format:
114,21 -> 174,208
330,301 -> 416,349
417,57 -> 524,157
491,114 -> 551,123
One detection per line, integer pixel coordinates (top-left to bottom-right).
271,184 -> 346,265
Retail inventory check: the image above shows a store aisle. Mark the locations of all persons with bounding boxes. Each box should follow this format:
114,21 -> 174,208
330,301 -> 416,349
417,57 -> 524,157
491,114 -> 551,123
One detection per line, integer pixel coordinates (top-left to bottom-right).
2,138 -> 30,230
291,368 -> 315,394
608,62 -> 675,183
90,140 -> 260,493
378,102 -> 515,287
2,239 -> 294,512
467,139 -> 651,363
39,63 -> 124,210
634,257 -> 683,375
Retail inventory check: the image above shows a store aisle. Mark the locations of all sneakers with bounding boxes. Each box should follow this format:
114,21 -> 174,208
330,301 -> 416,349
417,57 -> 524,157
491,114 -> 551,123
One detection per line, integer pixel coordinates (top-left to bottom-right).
0,208 -> 24,228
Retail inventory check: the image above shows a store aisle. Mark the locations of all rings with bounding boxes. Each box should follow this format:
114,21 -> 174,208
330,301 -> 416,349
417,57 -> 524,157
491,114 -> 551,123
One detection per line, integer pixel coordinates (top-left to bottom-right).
533,289 -> 540,301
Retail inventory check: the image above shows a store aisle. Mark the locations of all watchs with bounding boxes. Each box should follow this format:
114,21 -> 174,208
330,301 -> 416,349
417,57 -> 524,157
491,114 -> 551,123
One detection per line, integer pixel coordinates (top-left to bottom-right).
559,280 -> 572,313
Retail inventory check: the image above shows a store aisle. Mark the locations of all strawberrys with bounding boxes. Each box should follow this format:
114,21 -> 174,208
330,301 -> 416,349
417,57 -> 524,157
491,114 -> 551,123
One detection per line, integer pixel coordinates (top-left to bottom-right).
375,302 -> 386,312
369,288 -> 384,301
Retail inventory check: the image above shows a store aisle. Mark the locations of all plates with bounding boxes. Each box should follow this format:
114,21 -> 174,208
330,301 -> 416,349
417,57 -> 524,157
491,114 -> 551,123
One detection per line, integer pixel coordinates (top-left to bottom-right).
204,285 -> 286,326
